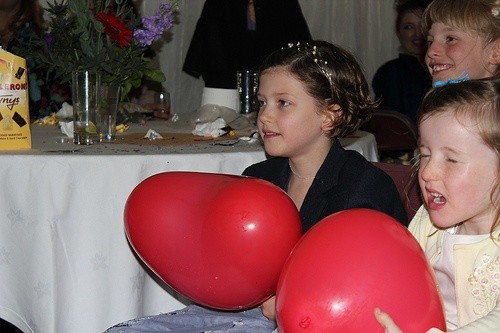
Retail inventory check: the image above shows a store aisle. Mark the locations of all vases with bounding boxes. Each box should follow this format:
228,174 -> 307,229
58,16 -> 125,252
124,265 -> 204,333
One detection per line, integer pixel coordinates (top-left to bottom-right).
71,70 -> 120,145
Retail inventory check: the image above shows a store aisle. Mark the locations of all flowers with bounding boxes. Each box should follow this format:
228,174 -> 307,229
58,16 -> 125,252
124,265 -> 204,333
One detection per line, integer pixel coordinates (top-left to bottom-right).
14,0 -> 180,125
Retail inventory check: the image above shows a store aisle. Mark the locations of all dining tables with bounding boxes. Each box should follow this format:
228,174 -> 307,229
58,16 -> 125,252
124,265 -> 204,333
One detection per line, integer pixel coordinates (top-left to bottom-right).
0,120 -> 380,333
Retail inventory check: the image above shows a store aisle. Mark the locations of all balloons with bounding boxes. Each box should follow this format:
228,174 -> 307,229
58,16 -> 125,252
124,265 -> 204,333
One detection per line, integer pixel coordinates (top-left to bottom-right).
275,208 -> 446,333
124,171 -> 302,312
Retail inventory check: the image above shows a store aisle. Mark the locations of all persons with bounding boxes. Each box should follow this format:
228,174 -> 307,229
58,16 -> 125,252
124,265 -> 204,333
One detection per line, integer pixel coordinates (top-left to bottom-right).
371,2 -> 429,109
374,75 -> 499,333
425,0 -> 500,88
103,40 -> 409,333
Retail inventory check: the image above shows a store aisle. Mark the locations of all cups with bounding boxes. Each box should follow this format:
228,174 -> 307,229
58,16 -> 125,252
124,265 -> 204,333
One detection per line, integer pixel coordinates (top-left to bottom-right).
153,91 -> 170,121
236,71 -> 260,117
71,71 -> 101,146
